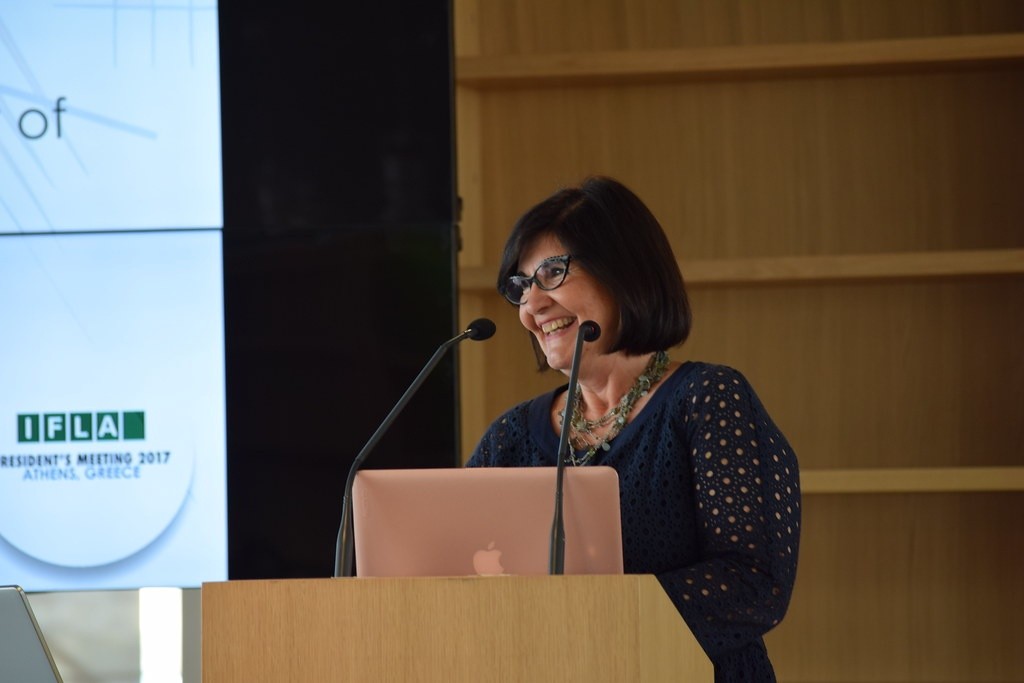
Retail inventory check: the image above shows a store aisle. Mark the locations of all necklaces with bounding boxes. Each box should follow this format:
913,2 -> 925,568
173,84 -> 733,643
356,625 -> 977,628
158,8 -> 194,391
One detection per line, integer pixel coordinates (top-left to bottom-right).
559,351 -> 672,467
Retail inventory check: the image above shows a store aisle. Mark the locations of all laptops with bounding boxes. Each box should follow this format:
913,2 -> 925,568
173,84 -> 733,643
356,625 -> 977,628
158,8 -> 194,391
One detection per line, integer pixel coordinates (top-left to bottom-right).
351,467 -> 626,577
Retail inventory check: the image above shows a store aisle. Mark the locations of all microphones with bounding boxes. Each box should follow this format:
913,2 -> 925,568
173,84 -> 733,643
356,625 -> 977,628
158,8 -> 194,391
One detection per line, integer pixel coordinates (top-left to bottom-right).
548,320 -> 602,575
334,318 -> 497,579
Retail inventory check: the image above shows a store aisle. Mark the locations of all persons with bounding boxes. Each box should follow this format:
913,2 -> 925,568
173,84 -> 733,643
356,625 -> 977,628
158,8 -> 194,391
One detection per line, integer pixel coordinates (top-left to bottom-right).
467,178 -> 801,683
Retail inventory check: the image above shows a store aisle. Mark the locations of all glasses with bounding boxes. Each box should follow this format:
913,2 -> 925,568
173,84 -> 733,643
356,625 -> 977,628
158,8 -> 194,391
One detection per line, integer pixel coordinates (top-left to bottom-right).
503,251 -> 586,305
